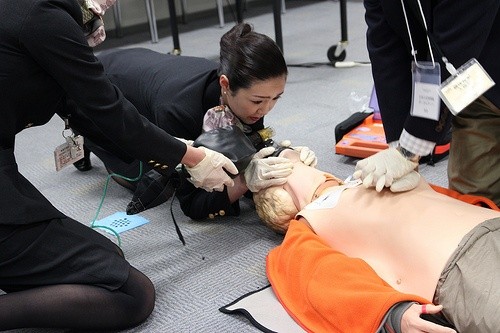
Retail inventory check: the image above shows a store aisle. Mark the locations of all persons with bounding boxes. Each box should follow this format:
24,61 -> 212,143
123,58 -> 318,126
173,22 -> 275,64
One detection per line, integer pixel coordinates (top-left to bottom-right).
0,0 -> 238,333
72,24 -> 318,222
252,148 -> 500,333
352,0 -> 500,210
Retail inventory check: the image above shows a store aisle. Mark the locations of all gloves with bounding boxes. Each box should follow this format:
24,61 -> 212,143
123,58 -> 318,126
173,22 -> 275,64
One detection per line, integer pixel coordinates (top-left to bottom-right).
244,146 -> 293,192
280,140 -> 317,167
354,147 -> 419,187
184,146 -> 239,192
353,170 -> 420,192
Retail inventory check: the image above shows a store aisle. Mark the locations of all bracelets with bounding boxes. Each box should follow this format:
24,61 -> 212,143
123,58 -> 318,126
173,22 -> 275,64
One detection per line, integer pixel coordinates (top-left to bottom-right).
396,143 -> 420,162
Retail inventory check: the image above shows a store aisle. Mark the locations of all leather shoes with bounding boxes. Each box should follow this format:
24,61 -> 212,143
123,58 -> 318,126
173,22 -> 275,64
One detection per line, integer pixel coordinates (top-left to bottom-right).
73,144 -> 92,172
